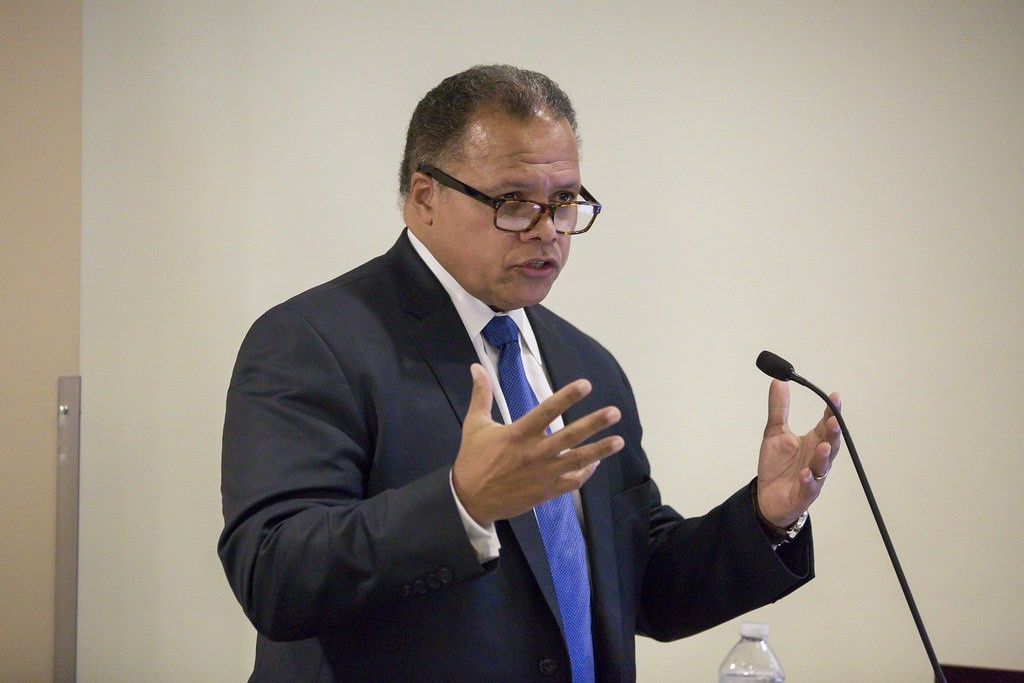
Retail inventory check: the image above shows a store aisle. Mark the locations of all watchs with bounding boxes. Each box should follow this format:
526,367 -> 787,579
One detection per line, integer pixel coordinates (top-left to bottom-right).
754,483 -> 808,541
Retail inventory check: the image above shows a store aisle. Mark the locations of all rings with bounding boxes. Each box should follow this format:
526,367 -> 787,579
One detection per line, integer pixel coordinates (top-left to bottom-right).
814,470 -> 828,481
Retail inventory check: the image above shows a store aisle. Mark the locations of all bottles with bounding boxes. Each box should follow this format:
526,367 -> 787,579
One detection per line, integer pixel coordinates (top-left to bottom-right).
718,621 -> 785,683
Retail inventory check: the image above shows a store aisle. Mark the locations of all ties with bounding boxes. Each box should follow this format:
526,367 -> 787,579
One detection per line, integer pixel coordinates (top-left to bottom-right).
482,314 -> 598,683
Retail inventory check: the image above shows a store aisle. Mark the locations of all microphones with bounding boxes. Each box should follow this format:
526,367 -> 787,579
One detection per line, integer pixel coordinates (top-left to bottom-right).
756,350 -> 947,683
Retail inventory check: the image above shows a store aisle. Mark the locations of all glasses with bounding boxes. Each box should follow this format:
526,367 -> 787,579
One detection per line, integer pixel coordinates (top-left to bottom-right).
417,162 -> 602,236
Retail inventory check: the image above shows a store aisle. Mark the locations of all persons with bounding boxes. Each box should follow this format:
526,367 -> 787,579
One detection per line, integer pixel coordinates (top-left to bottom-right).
215,67 -> 843,683
